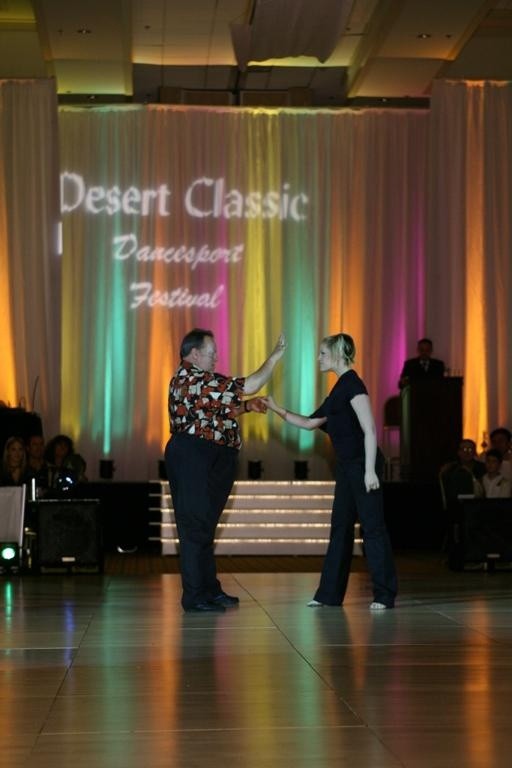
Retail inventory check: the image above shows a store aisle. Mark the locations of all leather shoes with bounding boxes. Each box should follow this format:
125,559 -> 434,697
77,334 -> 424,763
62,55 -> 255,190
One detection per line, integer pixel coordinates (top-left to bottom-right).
185,600 -> 226,613
211,594 -> 240,606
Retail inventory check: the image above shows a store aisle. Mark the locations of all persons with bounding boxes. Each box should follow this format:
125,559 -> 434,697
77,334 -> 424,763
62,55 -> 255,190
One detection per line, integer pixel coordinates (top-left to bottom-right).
459,438 -> 487,478
1,433 -> 85,500
164,327 -> 286,613
398,336 -> 445,390
174,609 -> 233,767
259,332 -> 399,612
489,426 -> 511,478
314,605 -> 402,767
480,448 -> 512,496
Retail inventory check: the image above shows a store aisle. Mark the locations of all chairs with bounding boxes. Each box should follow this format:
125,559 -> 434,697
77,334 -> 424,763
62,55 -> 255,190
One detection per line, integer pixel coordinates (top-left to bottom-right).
436,461 -> 500,575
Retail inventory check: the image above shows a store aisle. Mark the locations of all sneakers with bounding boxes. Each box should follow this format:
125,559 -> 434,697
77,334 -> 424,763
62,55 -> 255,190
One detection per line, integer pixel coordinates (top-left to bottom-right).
368,601 -> 387,610
306,599 -> 327,608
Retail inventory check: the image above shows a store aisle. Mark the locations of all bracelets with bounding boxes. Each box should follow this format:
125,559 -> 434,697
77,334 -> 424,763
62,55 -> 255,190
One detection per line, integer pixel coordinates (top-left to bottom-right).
243,400 -> 251,412
